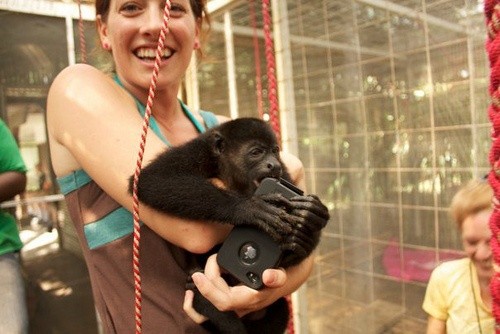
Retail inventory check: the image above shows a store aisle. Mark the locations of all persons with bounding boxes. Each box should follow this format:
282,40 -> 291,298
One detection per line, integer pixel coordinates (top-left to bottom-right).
46,0 -> 316,334
422,180 -> 500,333
0,119 -> 30,334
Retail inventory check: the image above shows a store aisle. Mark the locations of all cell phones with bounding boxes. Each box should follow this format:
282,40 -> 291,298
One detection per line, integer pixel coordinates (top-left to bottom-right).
216,177 -> 304,289
